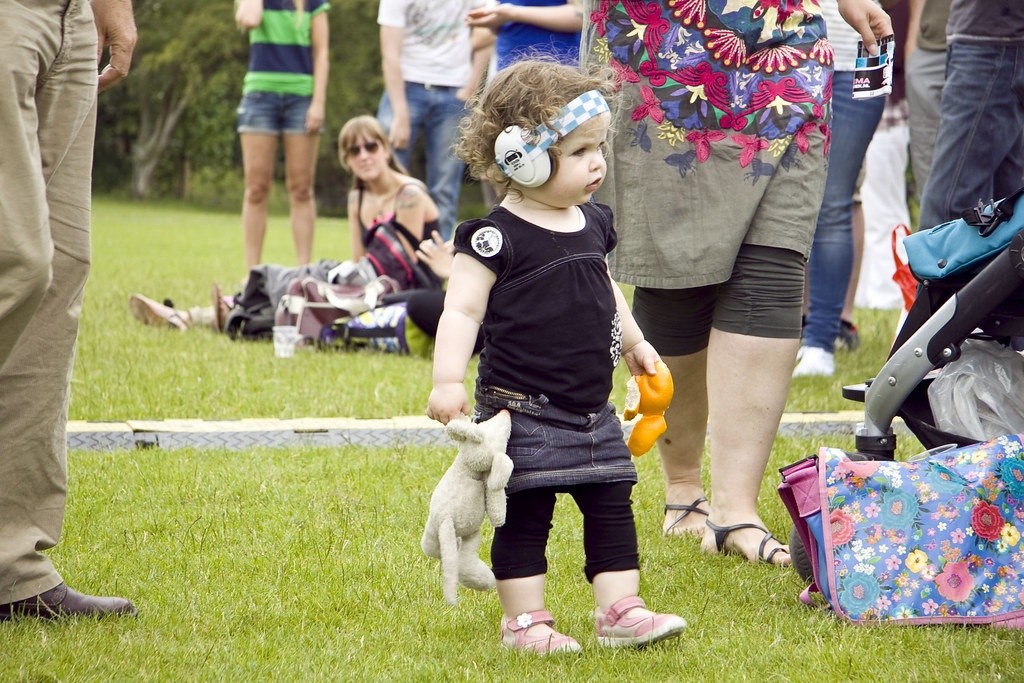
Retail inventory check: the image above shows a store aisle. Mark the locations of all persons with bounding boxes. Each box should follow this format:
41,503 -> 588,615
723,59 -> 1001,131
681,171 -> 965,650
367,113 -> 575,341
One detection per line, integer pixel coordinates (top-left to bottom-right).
579,0 -> 894,565
0,0 -> 136,625
426,59 -> 686,657
129,0 -> 1024,376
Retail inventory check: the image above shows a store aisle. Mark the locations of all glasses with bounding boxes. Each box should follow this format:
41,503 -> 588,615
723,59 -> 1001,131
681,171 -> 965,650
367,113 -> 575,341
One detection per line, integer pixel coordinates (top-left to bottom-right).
346,141 -> 378,155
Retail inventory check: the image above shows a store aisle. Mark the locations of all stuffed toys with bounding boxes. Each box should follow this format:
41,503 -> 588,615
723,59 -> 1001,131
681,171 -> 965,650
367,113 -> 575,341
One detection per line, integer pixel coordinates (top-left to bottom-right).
421,409 -> 515,608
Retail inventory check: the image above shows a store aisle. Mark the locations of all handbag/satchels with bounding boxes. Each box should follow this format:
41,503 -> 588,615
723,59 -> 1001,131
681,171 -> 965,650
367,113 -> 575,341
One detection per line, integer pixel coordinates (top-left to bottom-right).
774,432 -> 1024,631
317,294 -> 436,359
271,274 -> 402,348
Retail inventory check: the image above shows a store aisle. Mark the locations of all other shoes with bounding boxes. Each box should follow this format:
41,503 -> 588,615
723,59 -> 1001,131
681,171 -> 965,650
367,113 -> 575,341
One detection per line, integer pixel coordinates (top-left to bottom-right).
791,345 -> 835,377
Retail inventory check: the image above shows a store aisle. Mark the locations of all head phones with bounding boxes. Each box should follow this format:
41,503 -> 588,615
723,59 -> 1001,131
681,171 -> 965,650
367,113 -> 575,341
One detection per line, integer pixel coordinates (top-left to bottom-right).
495,90 -> 608,189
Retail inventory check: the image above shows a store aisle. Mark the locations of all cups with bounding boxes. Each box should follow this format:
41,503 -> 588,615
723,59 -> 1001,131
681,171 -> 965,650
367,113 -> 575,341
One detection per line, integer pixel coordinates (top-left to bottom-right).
272,325 -> 299,359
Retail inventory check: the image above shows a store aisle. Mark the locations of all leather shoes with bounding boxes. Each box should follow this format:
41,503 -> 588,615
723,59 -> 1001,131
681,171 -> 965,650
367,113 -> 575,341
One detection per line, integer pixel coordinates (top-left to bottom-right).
0,581 -> 134,621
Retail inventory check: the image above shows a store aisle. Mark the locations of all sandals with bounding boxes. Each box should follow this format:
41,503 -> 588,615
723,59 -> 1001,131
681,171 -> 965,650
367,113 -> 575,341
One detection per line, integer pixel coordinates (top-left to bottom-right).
663,495 -> 709,537
499,609 -> 580,657
128,292 -> 200,331
704,517 -> 792,568
211,285 -> 237,332
593,595 -> 689,649
